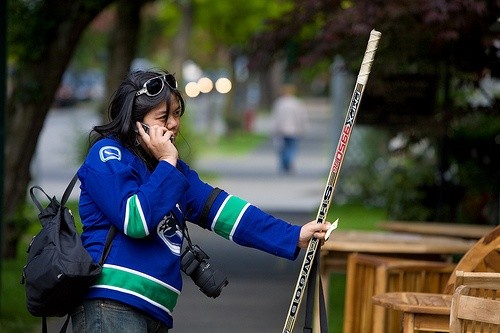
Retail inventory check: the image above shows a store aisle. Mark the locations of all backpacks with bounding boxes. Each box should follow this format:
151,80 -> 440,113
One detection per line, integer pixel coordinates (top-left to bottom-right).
21,133 -> 144,333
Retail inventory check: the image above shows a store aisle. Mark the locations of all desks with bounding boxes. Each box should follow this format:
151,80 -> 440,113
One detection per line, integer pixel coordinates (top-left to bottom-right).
308,222 -> 500,333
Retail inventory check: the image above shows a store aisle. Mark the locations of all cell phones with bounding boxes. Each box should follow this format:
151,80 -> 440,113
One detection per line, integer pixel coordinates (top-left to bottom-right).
132,121 -> 175,144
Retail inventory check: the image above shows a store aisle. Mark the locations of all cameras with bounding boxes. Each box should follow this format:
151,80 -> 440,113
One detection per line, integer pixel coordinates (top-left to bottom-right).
181,244 -> 229,299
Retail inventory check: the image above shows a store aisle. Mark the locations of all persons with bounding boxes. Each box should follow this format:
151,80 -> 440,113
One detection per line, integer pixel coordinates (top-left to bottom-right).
271,84 -> 310,177
72,67 -> 330,333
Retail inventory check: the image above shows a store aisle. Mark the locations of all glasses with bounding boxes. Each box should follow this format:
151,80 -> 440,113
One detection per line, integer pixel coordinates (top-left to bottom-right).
135,74 -> 178,97
